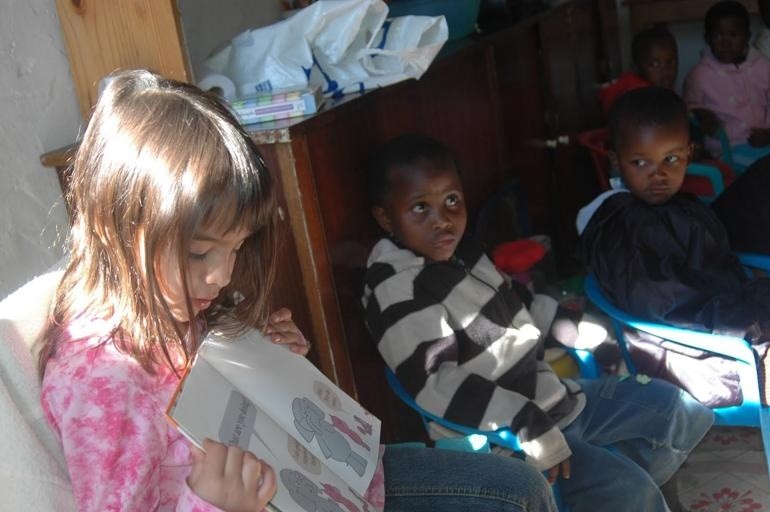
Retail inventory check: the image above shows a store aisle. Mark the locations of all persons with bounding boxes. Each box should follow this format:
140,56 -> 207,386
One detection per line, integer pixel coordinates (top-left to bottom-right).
358,133 -> 714,512
39,69 -> 558,512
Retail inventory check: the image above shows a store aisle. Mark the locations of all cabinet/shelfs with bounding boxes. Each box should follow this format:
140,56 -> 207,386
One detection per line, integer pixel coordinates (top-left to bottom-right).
42,0 -> 624,447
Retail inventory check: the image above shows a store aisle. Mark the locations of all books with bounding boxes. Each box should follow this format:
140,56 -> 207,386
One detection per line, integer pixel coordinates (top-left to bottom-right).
166,311 -> 382,511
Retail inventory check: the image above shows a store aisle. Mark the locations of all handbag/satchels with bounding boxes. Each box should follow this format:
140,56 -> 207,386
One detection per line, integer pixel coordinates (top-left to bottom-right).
196,0 -> 449,99
